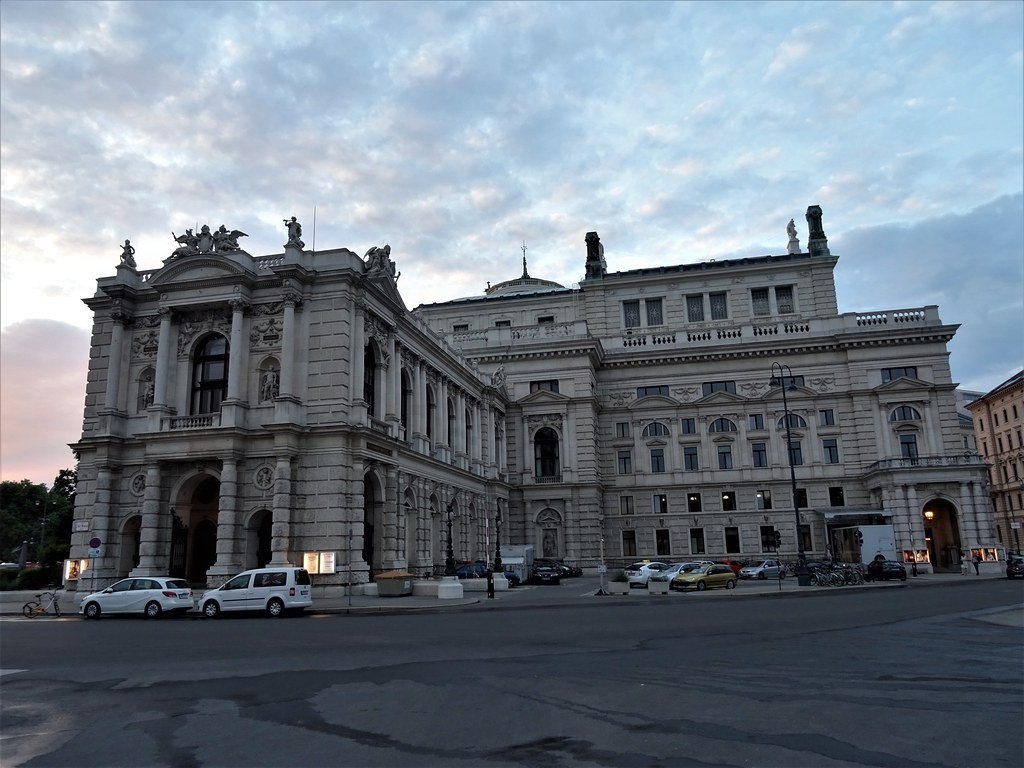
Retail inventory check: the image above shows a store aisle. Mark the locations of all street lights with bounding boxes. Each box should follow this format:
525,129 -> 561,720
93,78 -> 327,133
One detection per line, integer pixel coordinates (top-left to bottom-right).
34,499 -> 57,568
769,361 -> 811,586
992,476 -> 1024,558
495,517 -> 503,571
445,509 -> 457,576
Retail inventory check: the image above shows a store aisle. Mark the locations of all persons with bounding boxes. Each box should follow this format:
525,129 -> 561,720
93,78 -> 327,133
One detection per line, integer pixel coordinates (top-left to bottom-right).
375,242 -> 393,275
119,239 -> 135,265
960,553 -> 968,575
987,552 -> 995,560
143,375 -> 154,410
285,216 -> 305,249
786,218 -> 797,239
971,550 -> 981,576
262,364 -> 276,400
196,225 -> 214,254
167,228 -> 198,259
214,226 -> 240,250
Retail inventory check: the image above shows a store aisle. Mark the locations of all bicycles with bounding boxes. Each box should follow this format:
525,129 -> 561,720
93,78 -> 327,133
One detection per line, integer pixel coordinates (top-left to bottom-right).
809,562 -> 864,587
23,586 -> 63,618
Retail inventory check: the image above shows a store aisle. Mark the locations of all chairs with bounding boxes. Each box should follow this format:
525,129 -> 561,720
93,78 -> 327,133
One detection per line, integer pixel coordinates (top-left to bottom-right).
137,580 -> 146,589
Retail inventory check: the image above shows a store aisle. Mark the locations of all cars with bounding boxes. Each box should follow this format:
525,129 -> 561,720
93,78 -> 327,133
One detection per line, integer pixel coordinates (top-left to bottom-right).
78,577 -> 194,620
619,559 -> 743,592
673,564 -> 737,592
197,567 -> 313,617
531,559 -> 583,585
456,562 -> 520,588
864,560 -> 906,583
1006,558 -> 1024,580
738,559 -> 786,580
